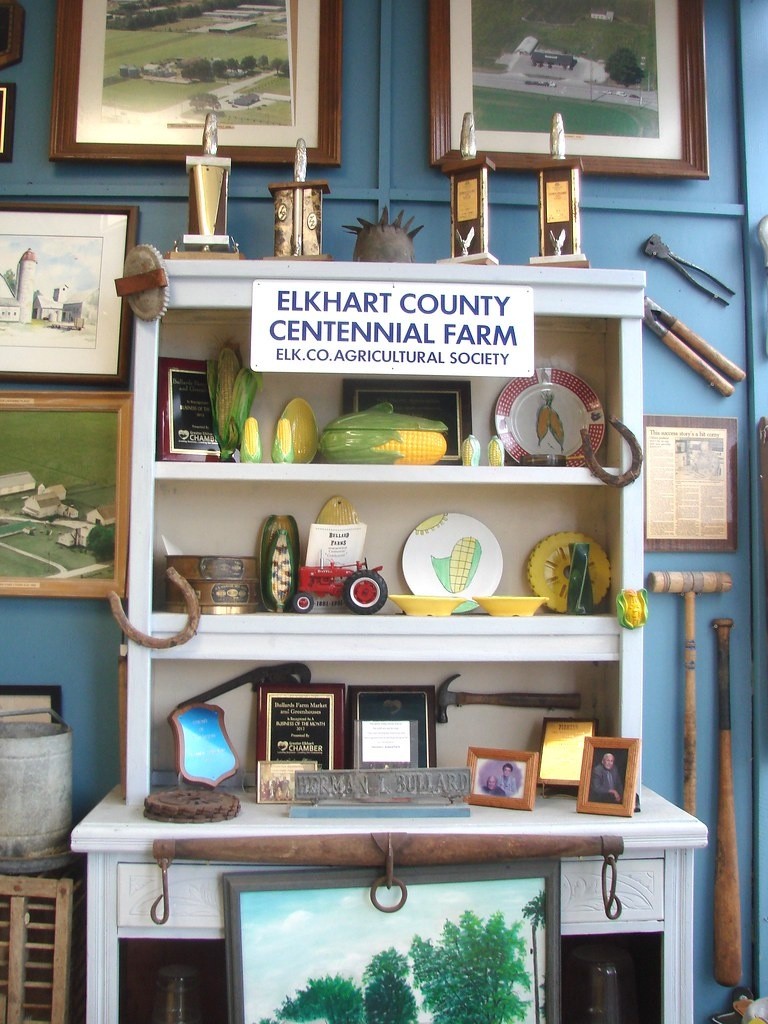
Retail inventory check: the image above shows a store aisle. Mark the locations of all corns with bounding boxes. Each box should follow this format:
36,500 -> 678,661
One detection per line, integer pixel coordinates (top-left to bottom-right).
461,434 -> 504,467
268,530 -> 293,612
319,403 -> 448,464
214,342 -> 295,463
615,588 -> 649,630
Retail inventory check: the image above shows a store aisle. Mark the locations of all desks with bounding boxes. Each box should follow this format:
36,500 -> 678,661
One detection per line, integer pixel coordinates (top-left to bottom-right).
71,783 -> 707,1024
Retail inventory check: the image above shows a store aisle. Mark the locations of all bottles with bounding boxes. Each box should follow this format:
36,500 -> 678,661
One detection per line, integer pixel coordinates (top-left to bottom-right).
568,543 -> 593,615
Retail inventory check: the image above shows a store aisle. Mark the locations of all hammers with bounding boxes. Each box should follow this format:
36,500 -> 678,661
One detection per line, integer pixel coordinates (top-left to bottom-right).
437,674 -> 582,724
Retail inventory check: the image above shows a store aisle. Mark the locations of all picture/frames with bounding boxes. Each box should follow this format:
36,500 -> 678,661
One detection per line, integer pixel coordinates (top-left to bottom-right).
430,0 -> 711,179
464,748 -> 539,811
577,735 -> 640,819
219,860 -> 563,1023
49,0 -> 342,167
0,391 -> 130,598
0,202 -> 140,385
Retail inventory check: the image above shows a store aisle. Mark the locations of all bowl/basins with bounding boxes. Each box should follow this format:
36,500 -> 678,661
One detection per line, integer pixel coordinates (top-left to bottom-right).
387,595 -> 467,616
472,596 -> 550,617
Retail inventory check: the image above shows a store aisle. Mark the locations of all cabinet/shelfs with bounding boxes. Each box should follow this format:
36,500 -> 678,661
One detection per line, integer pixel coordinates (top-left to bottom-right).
116,261 -> 645,814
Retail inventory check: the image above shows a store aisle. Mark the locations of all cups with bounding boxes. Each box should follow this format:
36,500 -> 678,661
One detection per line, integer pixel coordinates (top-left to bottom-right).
521,454 -> 566,467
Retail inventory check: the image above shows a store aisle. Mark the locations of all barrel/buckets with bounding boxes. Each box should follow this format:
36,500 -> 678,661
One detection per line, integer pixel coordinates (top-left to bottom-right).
0,708 -> 73,873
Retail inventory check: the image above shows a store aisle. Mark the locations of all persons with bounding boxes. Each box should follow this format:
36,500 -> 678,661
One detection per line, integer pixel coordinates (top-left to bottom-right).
481,762 -> 518,796
589,753 -> 624,804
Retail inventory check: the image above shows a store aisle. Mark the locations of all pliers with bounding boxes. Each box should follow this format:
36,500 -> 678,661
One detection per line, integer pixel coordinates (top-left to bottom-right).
644,235 -> 736,305
642,294 -> 748,398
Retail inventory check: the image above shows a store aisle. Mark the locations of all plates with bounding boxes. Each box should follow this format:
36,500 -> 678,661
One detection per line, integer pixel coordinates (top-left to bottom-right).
402,514 -> 503,614
495,368 -> 605,466
